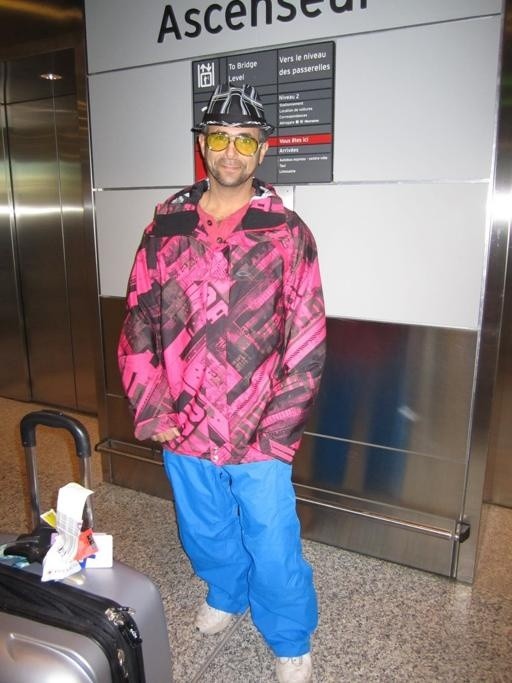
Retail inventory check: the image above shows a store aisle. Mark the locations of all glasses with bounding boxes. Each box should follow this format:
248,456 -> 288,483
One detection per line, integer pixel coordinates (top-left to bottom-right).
203,130 -> 266,157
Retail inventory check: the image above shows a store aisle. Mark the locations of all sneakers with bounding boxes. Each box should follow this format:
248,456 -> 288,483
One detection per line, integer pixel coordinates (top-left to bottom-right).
195,598 -> 234,635
276,652 -> 312,683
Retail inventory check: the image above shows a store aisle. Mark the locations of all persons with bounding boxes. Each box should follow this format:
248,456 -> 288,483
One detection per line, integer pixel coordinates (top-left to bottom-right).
293,318 -> 406,533
117,82 -> 328,683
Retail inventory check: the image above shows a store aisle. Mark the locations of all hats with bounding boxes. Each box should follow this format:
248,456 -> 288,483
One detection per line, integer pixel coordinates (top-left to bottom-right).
190,81 -> 274,137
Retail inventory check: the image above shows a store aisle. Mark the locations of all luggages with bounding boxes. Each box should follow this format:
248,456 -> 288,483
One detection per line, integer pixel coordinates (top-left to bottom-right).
0,528 -> 175,683
